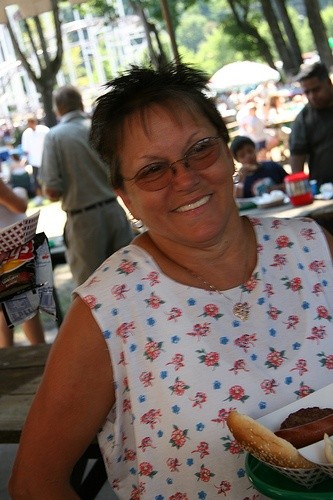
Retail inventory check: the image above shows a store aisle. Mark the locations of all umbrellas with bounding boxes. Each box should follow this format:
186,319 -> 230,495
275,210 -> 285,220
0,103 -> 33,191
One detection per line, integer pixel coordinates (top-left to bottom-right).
203,59 -> 282,95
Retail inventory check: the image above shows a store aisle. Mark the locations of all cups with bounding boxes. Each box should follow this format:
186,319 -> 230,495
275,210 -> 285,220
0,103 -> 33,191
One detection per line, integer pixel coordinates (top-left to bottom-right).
284,171 -> 313,207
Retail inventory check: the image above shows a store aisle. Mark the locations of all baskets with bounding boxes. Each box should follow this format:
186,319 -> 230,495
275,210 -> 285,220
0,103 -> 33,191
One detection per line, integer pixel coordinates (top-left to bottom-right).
251,454 -> 323,489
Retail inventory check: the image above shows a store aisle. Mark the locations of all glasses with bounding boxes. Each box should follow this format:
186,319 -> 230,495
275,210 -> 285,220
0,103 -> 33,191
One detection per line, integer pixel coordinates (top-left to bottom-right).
120,135 -> 223,191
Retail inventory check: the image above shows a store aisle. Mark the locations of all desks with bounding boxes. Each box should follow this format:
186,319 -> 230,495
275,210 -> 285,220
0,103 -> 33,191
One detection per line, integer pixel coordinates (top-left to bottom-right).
0,345 -> 108,500
233,197 -> 333,221
44,236 -> 68,329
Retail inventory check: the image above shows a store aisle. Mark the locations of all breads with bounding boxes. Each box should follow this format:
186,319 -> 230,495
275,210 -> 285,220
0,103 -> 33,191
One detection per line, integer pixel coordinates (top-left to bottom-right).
225,411 -> 313,468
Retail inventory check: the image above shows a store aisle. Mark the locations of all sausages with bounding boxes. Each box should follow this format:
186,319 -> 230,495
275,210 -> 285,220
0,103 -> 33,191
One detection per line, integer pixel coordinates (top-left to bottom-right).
274,412 -> 333,449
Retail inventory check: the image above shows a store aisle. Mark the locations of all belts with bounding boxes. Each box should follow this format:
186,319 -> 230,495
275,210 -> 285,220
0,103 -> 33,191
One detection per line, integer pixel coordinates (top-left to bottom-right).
68,198 -> 117,215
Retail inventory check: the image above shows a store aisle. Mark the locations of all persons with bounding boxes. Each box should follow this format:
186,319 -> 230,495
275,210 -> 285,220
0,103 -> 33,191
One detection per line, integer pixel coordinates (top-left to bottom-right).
285,60 -> 333,251
7,72 -> 333,499
37,87 -> 135,289
0,77 -> 310,206
0,176 -> 49,346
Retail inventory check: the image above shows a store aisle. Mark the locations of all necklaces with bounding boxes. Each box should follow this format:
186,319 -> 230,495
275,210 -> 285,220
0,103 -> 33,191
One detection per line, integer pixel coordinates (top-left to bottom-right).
148,224 -> 253,322
